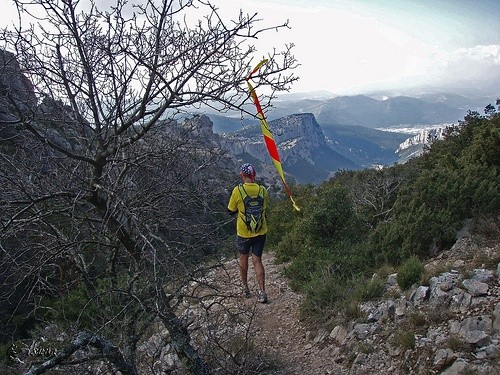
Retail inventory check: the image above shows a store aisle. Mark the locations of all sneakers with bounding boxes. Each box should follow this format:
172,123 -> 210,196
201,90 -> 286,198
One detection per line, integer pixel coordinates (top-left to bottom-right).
257,292 -> 267,302
243,288 -> 250,298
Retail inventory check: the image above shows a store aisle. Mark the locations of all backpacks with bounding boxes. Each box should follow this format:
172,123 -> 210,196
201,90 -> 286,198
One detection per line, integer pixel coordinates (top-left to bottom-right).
238,183 -> 265,233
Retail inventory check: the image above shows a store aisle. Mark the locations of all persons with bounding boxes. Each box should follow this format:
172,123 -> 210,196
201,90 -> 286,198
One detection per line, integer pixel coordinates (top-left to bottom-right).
227,164 -> 269,303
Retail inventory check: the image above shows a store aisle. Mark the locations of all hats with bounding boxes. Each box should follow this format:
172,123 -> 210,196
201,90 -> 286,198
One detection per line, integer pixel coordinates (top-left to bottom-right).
240,163 -> 256,179
